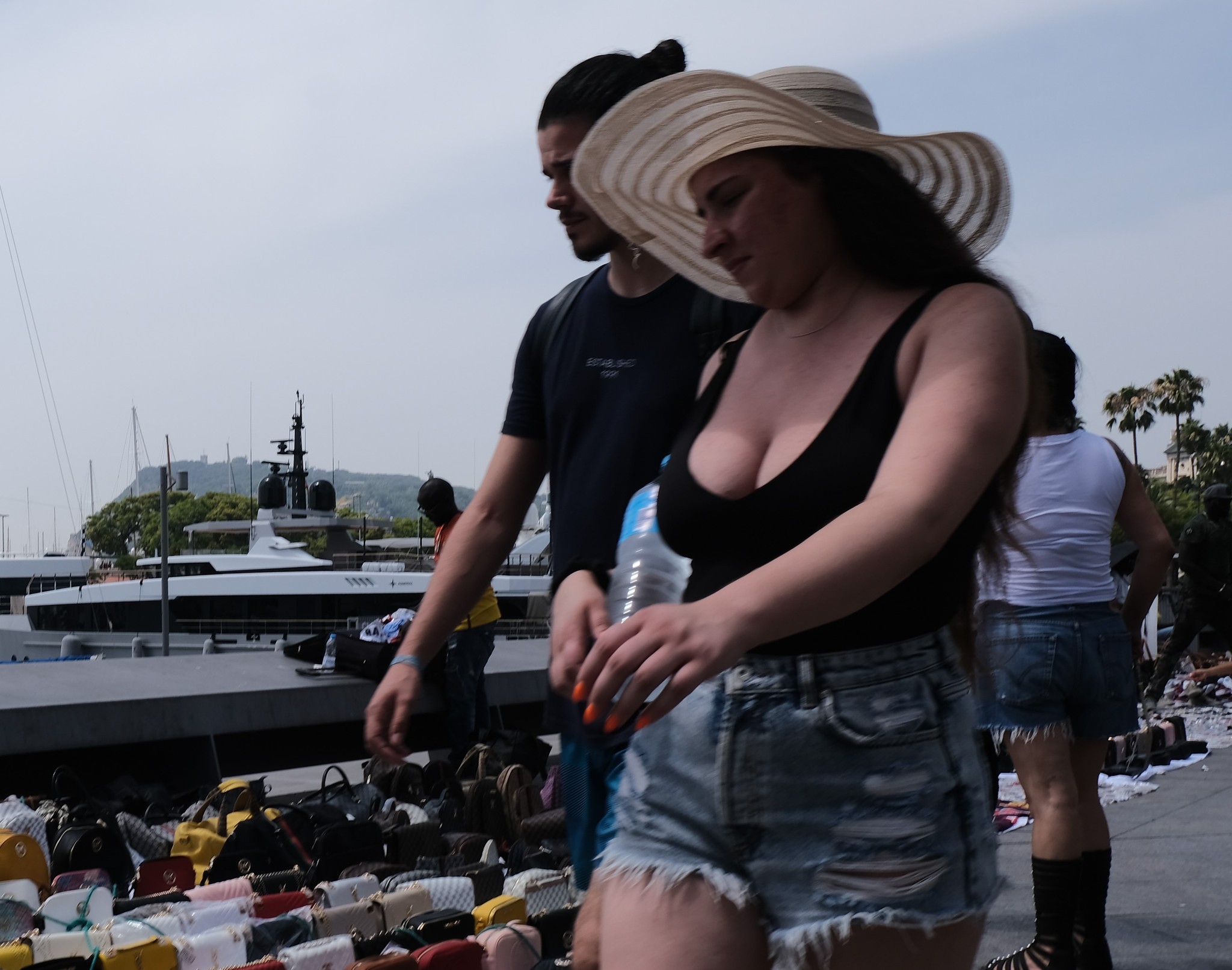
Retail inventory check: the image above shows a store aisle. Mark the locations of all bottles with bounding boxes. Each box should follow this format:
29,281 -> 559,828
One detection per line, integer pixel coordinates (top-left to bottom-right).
321,633 -> 337,673
605,455 -> 693,705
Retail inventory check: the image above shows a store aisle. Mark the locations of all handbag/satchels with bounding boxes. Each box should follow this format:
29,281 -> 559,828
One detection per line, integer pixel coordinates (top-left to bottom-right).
1140,638 -> 1155,682
0,742 -> 583,970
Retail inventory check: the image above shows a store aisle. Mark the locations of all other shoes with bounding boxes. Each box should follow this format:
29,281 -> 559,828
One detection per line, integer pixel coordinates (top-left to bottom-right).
1187,695 -> 1225,708
1144,697 -> 1158,712
1164,693 -> 1176,706
1179,681 -> 1202,700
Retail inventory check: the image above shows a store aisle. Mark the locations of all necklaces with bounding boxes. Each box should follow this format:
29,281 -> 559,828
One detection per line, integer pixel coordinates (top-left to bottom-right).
780,265 -> 868,340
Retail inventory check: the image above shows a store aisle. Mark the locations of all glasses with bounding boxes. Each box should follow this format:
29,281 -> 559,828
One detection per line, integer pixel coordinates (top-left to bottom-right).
417,505 -> 438,516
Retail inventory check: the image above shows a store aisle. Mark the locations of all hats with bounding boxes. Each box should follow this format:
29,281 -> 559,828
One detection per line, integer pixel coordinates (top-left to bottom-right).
571,66 -> 1012,306
1200,483 -> 1232,498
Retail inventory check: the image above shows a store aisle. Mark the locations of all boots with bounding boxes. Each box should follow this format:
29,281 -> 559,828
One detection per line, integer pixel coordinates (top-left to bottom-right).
977,846 -> 1114,970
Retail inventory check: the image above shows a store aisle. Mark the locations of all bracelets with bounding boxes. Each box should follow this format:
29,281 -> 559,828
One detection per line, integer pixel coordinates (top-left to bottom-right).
391,654 -> 425,670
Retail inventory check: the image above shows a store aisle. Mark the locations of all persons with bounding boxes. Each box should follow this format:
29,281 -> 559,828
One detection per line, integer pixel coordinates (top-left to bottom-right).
542,65 -> 1032,969
416,477 -> 503,770
1109,481 -> 1232,714
961,329 -> 1172,969
364,36 -> 766,883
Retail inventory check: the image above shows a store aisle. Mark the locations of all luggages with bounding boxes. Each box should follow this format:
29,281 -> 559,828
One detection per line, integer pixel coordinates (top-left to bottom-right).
283,623 -> 449,685
